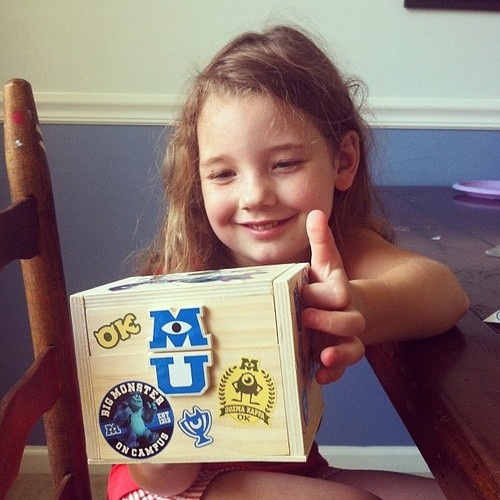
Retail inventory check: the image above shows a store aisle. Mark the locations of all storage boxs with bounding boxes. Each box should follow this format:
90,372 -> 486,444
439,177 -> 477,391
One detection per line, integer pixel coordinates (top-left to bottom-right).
69,262 -> 324,464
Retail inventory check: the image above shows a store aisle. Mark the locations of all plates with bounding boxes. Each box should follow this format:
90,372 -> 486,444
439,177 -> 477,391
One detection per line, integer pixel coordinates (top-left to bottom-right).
452,181 -> 500,200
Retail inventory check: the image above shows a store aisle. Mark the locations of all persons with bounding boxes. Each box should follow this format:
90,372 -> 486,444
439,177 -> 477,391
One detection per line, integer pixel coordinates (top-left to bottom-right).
106,24 -> 471,500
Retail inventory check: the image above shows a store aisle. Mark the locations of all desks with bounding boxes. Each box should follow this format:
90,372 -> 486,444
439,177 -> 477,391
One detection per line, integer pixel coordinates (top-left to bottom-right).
365,184 -> 500,500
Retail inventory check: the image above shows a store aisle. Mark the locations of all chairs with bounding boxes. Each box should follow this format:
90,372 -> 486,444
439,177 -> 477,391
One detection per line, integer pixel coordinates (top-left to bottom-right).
0,78 -> 95,499
